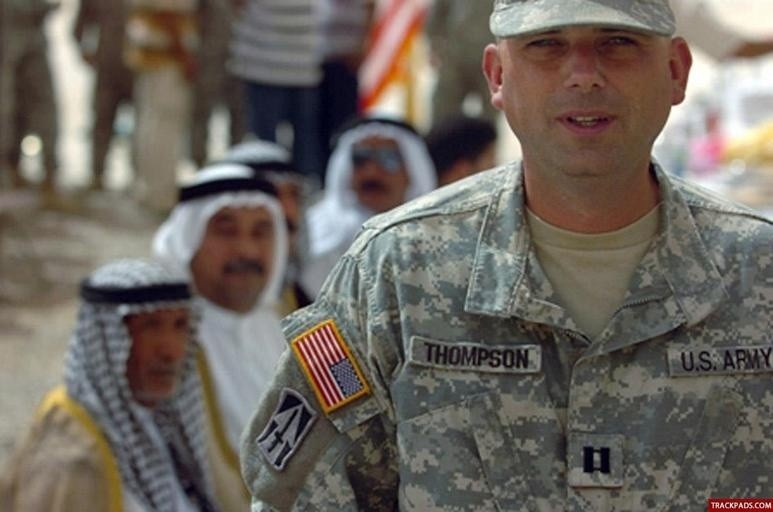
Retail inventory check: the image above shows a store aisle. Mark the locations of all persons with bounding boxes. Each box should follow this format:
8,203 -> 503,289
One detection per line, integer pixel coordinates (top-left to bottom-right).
1,0 -> 511,212
1,112 -> 497,511
239,1 -> 771,512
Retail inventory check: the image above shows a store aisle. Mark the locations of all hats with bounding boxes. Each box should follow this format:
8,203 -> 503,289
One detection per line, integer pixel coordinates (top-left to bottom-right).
488,0 -> 678,36
